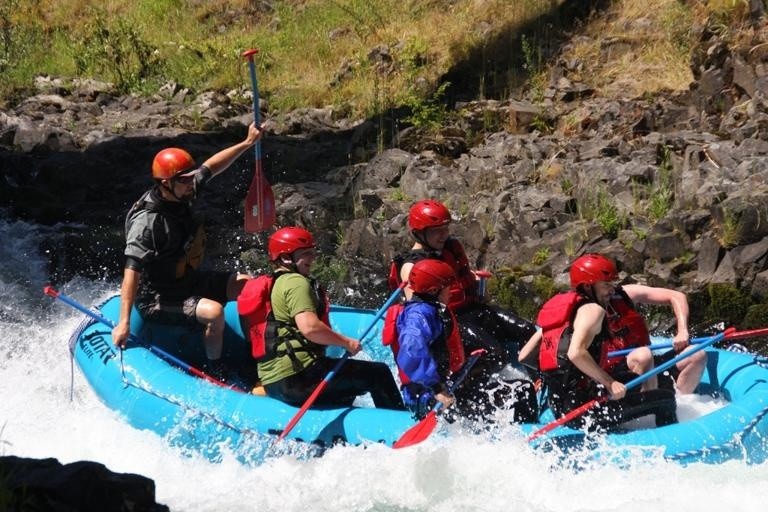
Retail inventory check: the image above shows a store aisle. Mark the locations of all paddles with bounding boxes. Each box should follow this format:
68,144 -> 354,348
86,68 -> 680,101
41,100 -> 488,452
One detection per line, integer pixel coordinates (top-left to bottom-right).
44,287 -> 247,392
278,281 -> 407,443
393,349 -> 486,448
527,326 -> 736,441
244,49 -> 276,233
607,327 -> 768,357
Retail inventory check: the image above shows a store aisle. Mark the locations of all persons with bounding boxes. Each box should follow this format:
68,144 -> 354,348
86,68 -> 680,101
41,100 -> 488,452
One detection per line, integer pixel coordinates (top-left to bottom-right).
518,254 -> 676,435
247,226 -> 405,412
385,259 -> 538,425
595,284 -> 708,396
390,200 -> 536,360
111,122 -> 267,375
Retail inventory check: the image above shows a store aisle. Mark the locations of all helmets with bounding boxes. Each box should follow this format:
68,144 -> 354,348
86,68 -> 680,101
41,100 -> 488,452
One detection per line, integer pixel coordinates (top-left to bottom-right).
410,259 -> 456,295
153,147 -> 202,180
568,253 -> 614,287
409,200 -> 451,242
268,227 -> 313,261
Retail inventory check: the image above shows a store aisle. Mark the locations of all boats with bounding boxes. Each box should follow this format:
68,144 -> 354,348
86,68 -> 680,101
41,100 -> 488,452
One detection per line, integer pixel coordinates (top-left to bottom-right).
66,295 -> 768,473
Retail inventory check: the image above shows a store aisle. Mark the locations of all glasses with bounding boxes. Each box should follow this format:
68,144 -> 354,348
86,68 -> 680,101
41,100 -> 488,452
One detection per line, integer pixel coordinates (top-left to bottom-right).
178,174 -> 196,183
294,247 -> 317,260
